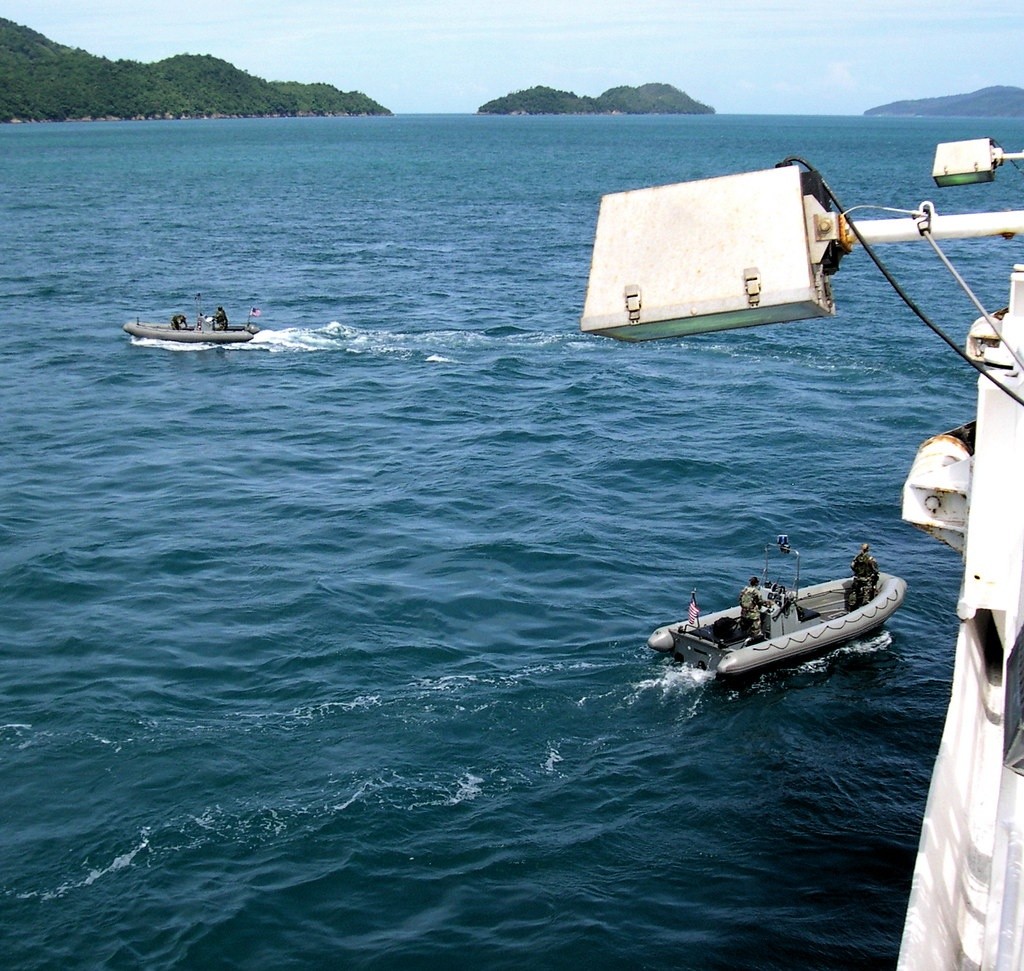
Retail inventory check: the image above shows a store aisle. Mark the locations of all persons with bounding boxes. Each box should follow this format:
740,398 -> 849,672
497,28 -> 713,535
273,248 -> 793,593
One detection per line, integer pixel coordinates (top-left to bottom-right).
739,577 -> 765,646
847,543 -> 880,613
170,314 -> 187,331
213,305 -> 228,331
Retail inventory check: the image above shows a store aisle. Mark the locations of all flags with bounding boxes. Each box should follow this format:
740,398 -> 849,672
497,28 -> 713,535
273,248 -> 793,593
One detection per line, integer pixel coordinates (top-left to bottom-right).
251,307 -> 261,317
688,596 -> 700,624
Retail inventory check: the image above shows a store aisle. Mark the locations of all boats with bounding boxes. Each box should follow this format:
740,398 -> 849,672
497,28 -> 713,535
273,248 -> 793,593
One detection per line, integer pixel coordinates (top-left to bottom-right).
577,134 -> 1022,971
646,571 -> 908,685
123,319 -> 262,344
